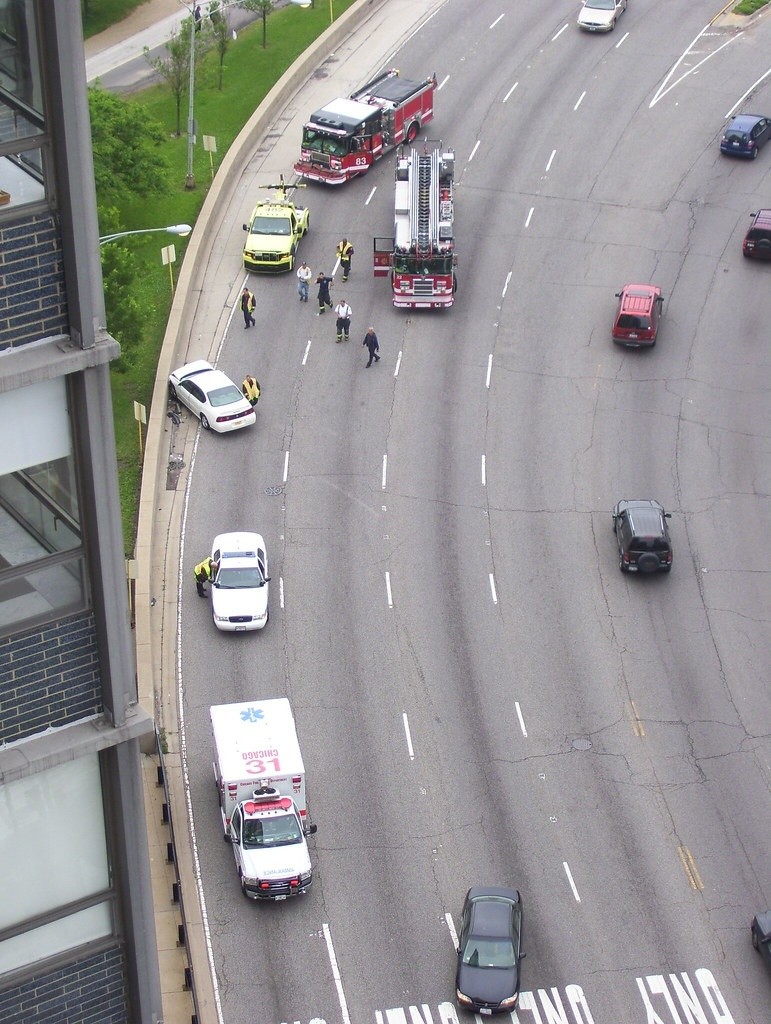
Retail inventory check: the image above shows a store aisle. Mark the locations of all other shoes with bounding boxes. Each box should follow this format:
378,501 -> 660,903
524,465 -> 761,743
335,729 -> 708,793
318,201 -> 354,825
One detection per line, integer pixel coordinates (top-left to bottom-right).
375,356 -> 380,361
305,297 -> 308,302
336,334 -> 342,342
342,276 -> 348,283
366,364 -> 370,368
329,300 -> 333,308
244,326 -> 250,329
252,318 -> 255,326
300,295 -> 304,301
318,307 -> 325,315
345,335 -> 349,341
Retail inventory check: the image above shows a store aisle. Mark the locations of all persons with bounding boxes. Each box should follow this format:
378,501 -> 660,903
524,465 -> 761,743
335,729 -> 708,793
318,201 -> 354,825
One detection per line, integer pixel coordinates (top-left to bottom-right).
296,261 -> 312,302
334,299 -> 352,343
362,327 -> 381,368
195,557 -> 218,598
314,271 -> 334,314
242,375 -> 261,407
240,287 -> 257,329
336,238 -> 354,282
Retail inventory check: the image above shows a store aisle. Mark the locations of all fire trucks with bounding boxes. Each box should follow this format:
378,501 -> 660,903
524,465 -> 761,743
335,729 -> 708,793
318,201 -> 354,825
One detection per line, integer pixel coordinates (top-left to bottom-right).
292,68 -> 438,186
373,137 -> 457,310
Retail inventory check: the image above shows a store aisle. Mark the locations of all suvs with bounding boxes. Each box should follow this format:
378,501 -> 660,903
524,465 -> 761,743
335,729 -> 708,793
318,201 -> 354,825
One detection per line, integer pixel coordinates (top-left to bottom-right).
742,209 -> 771,262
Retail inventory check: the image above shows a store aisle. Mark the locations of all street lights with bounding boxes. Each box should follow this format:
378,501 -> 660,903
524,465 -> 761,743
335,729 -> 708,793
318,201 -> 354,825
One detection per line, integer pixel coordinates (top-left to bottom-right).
184,1 -> 312,190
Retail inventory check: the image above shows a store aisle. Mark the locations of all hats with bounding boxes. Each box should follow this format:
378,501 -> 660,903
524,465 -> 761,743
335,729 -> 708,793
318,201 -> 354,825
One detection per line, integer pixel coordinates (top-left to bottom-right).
302,261 -> 306,267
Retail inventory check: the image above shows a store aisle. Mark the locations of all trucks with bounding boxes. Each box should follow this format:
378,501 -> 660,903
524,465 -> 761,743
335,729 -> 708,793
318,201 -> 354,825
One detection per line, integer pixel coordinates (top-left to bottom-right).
243,175 -> 310,276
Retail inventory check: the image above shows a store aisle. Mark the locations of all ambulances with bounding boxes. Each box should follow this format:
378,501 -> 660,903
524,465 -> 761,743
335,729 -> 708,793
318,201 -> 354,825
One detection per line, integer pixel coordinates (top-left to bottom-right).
210,697 -> 317,904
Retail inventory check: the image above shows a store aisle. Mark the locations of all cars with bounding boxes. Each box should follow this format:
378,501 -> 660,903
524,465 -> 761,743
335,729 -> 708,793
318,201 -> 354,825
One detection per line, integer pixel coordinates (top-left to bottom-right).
576,0 -> 628,35
614,284 -> 664,350
212,532 -> 272,633
169,359 -> 257,433
720,113 -> 771,160
455,886 -> 527,1016
751,911 -> 771,976
